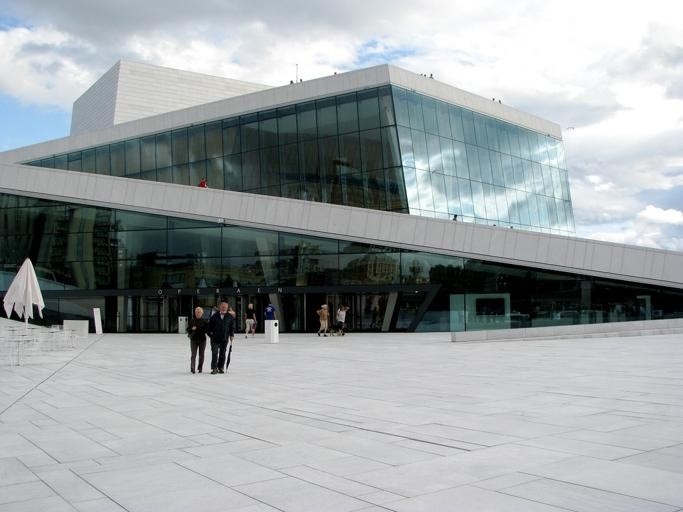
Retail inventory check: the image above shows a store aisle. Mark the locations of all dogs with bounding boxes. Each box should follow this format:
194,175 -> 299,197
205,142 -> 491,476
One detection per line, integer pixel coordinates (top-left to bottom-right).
327,320 -> 344,336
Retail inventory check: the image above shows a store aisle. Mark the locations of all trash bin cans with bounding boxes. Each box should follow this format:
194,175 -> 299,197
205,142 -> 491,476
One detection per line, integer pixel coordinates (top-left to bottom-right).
265,320 -> 279,344
178,316 -> 188,334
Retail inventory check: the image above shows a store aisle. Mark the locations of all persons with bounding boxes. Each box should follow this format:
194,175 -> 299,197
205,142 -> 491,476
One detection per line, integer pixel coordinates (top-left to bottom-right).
244,303 -> 257,339
186,302 -> 236,374
264,304 -> 277,320
334,303 -> 349,336
316,304 -> 329,337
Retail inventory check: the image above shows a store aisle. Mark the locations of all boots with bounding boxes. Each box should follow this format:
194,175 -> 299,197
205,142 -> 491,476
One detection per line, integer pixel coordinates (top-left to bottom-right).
342,333 -> 345,336
192,370 -> 202,374
211,369 -> 224,374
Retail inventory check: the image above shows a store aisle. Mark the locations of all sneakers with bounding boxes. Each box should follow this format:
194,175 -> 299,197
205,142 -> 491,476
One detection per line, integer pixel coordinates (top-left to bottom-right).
318,331 -> 329,337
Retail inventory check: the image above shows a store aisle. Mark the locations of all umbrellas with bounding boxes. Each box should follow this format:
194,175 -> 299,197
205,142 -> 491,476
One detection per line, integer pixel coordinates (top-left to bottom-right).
3,259 -> 45,324
